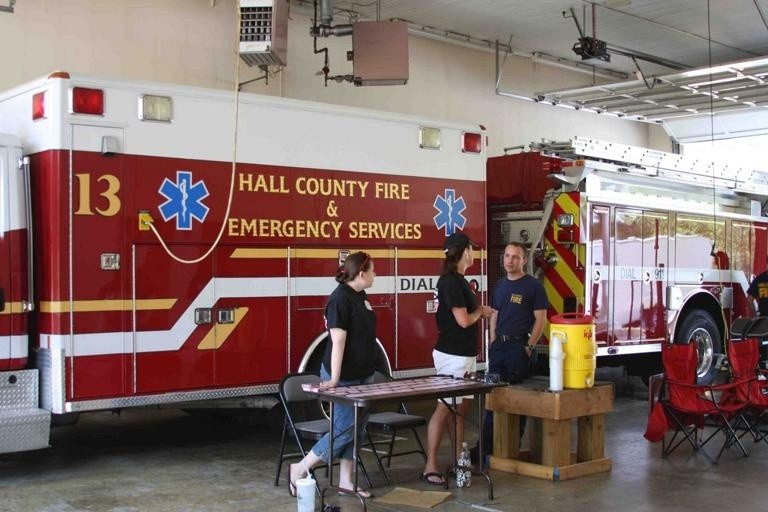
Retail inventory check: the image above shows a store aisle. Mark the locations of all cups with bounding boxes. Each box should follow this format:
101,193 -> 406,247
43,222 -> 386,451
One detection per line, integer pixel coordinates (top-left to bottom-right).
296,478 -> 316,511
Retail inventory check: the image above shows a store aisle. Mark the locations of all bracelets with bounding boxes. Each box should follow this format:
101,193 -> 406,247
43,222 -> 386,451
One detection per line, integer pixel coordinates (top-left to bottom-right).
525,344 -> 534,352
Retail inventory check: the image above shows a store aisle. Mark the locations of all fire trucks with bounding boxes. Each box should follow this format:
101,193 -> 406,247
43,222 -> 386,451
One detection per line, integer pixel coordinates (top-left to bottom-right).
487,135 -> 767,392
0,70 -> 487,454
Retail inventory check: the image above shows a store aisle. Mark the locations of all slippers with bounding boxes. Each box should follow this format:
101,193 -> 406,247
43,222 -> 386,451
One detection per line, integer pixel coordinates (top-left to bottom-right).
420,470 -> 448,486
335,483 -> 373,499
288,462 -> 299,498
447,465 -> 463,477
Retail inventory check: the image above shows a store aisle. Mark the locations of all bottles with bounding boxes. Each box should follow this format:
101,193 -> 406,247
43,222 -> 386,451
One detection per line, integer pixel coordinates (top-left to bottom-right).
457,440 -> 473,487
548,335 -> 564,393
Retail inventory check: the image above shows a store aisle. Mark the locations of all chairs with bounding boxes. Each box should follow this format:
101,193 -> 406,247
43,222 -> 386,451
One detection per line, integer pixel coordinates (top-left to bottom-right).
726,336 -> 768,447
654,339 -> 756,463
274,372 -> 372,497
361,368 -> 428,488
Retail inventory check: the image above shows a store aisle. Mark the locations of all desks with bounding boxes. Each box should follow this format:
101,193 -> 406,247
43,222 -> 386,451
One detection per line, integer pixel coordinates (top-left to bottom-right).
304,373 -> 509,512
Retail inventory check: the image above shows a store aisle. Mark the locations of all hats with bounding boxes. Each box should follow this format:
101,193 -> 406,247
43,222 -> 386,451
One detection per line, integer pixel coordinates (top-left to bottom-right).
442,232 -> 479,254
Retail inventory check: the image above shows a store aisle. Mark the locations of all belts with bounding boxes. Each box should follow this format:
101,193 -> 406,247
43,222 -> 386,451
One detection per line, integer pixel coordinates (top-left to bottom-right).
494,333 -> 529,344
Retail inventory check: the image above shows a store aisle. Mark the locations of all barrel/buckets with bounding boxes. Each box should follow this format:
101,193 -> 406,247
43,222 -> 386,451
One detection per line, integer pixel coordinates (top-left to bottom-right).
549,311 -> 598,390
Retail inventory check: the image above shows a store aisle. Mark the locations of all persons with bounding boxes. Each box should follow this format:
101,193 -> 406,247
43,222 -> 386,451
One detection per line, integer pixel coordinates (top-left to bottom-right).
469,242 -> 550,464
288,251 -> 377,498
420,233 -> 496,485
746,254 -> 768,318
